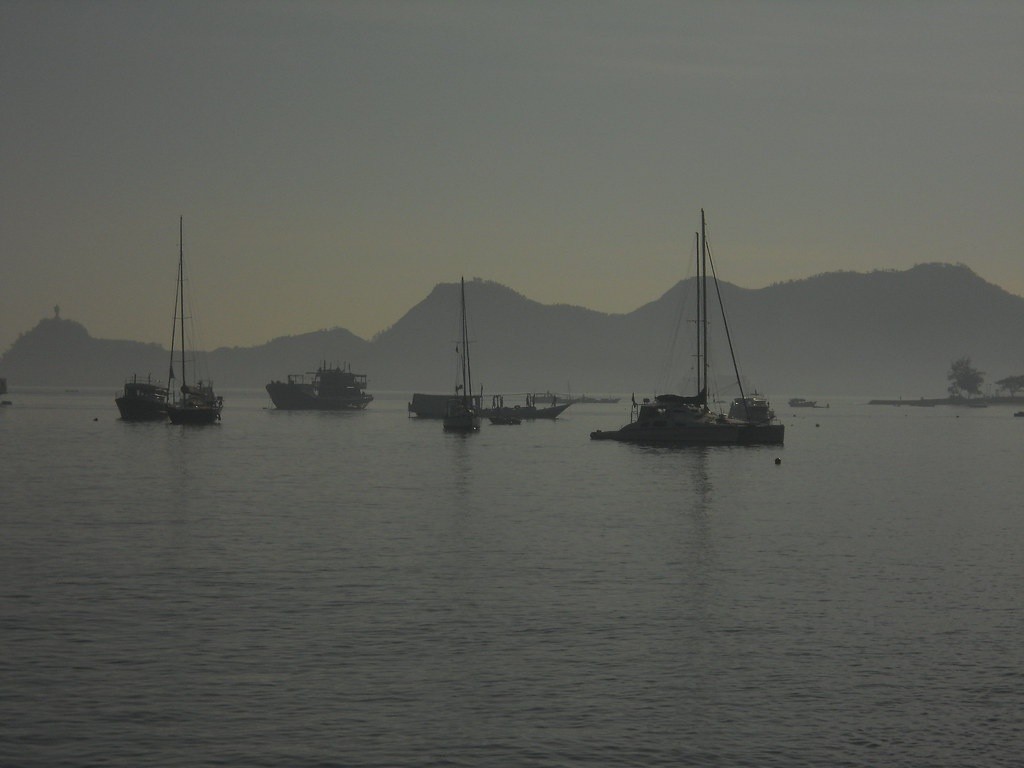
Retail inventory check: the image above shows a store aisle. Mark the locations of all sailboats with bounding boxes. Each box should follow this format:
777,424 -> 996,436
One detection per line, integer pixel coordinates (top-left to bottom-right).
443,277 -> 482,431
115,215 -> 224,425
591,209 -> 784,444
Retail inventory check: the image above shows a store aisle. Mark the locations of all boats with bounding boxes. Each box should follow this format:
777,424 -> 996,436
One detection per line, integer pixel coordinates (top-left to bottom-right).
788,398 -> 816,407
489,417 -> 522,424
409,394 -> 483,419
479,403 -> 572,419
265,361 -> 374,410
529,380 -> 621,403
729,390 -> 774,424
